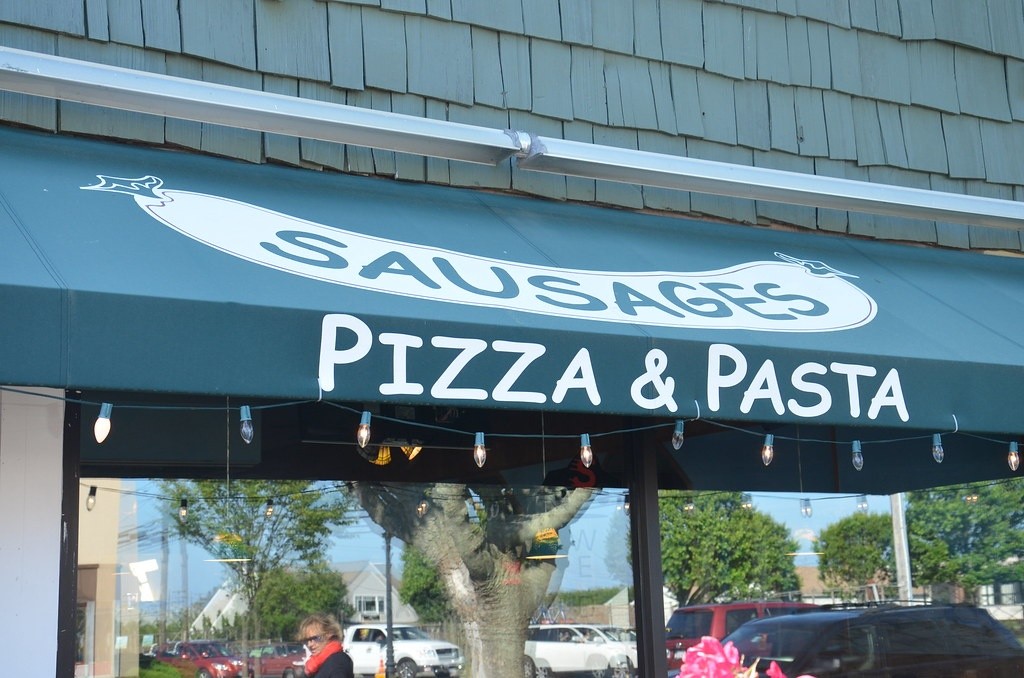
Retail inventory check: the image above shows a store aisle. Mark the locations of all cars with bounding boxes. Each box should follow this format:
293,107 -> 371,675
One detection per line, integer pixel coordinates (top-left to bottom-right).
140,597 -> 1024,678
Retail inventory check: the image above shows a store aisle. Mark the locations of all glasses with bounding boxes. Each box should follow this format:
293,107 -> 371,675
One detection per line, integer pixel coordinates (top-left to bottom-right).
303,633 -> 326,646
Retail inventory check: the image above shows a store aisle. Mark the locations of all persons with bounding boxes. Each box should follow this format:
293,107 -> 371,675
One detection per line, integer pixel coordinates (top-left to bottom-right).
559,628 -> 602,642
373,633 -> 384,642
298,610 -> 355,678
